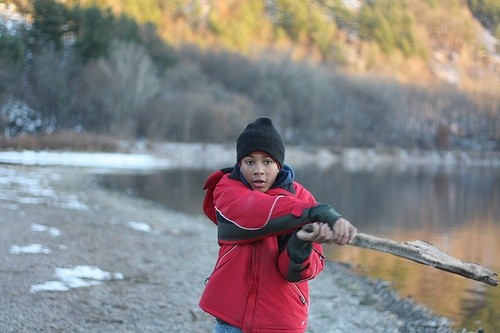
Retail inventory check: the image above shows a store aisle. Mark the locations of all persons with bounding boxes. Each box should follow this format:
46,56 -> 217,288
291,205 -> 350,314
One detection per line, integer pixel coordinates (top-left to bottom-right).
199,117 -> 358,333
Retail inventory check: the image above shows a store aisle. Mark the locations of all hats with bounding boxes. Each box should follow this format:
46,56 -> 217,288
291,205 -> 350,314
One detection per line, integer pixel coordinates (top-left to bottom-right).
236,117 -> 285,167
